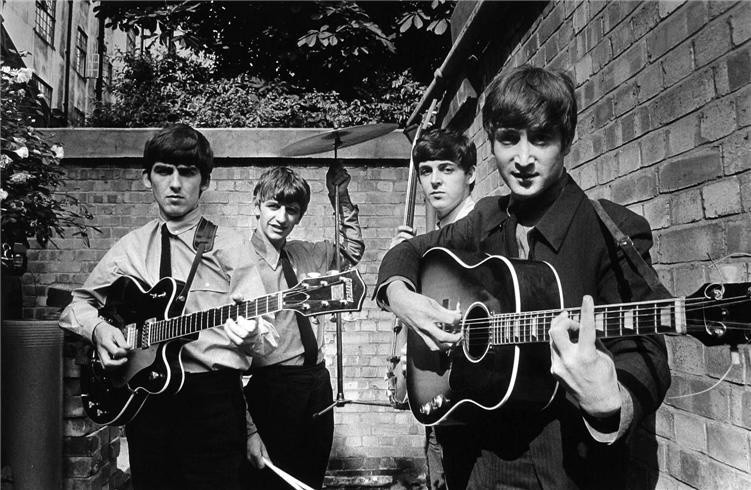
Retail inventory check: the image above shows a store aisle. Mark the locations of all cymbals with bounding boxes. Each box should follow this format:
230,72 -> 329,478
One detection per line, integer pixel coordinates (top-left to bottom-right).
279,123 -> 399,156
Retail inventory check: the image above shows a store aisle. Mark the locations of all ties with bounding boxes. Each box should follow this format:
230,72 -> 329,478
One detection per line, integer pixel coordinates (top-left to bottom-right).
278,249 -> 318,369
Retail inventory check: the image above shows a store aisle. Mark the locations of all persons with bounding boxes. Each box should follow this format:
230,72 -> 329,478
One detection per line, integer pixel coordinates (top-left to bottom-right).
241,159 -> 366,490
57,122 -> 280,490
389,126 -> 479,490
370,62 -> 671,490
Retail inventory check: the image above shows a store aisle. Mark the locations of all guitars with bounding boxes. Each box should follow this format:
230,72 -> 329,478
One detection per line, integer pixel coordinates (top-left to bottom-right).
405,246 -> 750,426
79,269 -> 367,427
